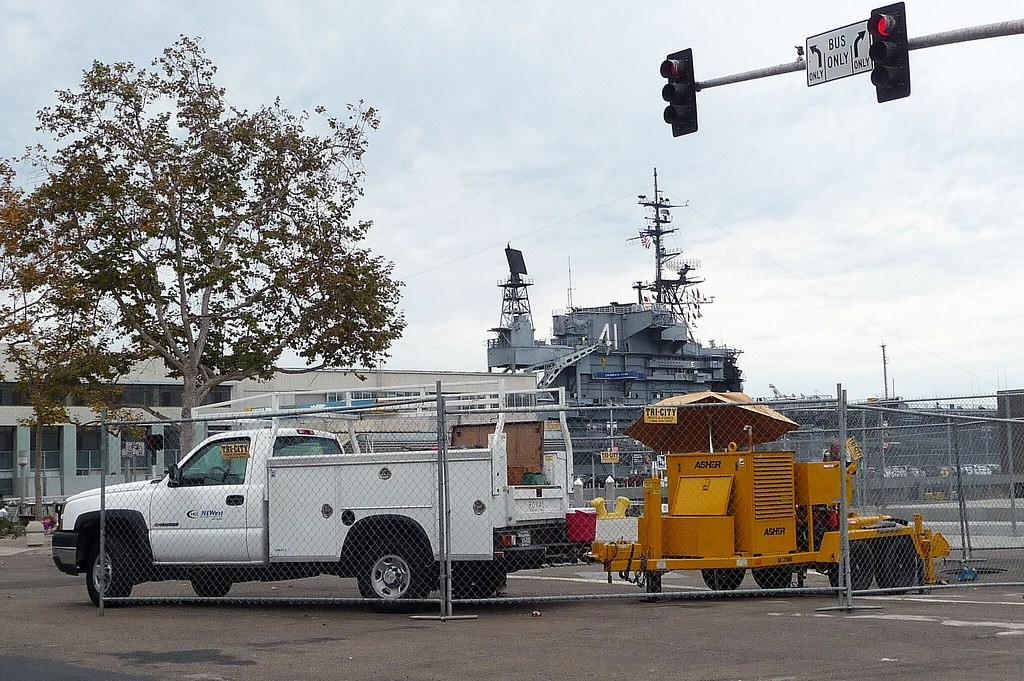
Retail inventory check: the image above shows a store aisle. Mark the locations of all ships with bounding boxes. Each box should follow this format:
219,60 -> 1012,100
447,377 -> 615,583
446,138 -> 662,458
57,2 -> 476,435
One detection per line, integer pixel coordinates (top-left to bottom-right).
478,168 -> 749,422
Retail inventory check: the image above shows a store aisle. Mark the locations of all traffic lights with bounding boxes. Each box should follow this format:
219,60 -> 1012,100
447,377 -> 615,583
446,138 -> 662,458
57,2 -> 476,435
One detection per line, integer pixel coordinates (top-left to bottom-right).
867,1 -> 913,107
660,48 -> 700,139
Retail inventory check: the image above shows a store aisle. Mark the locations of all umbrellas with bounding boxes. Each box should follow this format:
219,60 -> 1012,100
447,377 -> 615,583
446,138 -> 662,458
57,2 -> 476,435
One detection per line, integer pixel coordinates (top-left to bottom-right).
623,392 -> 800,452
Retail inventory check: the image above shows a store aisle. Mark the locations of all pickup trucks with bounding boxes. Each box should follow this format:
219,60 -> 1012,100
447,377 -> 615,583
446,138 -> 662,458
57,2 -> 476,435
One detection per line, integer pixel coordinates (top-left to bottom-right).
50,388 -> 600,612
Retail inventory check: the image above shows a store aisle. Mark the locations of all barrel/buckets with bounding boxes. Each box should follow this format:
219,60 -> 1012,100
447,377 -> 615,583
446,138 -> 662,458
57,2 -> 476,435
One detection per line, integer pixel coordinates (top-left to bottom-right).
26,520 -> 43,546
565,510 -> 599,541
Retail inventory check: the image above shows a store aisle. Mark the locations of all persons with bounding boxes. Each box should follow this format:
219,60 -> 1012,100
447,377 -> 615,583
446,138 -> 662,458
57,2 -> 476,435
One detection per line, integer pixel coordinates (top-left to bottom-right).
823,441 -> 841,461
625,471 -> 652,487
0,506 -> 9,517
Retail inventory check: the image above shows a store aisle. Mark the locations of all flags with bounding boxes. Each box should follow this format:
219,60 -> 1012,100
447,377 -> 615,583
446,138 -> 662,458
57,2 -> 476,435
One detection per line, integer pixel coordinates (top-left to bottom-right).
640,232 -> 651,249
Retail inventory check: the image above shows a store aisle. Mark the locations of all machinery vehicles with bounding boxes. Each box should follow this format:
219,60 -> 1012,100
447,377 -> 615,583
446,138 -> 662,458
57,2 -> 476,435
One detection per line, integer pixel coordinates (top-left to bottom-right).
589,425 -> 952,597
